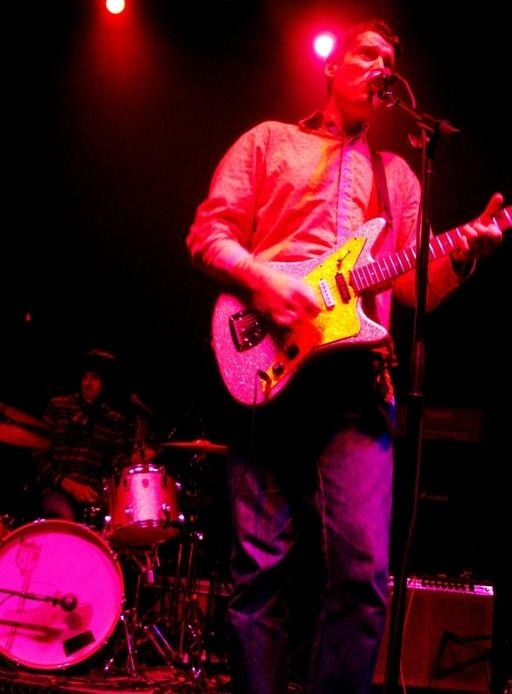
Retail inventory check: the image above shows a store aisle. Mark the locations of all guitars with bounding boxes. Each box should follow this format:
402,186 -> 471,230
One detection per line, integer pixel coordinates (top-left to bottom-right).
211,206 -> 512,405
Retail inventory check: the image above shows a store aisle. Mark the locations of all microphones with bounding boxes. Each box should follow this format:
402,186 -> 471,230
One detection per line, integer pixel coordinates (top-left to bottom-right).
368,68 -> 397,93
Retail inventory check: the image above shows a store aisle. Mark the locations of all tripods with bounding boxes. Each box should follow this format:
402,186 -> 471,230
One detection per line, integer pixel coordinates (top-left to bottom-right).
84,448 -> 239,694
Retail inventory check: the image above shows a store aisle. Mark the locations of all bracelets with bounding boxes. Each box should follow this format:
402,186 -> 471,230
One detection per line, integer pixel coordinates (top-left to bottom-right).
56,474 -> 65,489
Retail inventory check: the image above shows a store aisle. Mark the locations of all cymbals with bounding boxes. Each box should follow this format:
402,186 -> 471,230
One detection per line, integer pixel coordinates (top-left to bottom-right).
0,403 -> 47,430
0,423 -> 51,448
161,438 -> 226,450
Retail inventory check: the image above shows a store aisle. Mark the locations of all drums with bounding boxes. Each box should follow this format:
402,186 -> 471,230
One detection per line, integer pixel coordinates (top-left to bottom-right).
111,464 -> 181,547
1,518 -> 126,672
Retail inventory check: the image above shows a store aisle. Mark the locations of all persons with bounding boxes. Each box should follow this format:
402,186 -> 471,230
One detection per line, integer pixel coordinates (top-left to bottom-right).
189,14 -> 507,694
20,344 -> 132,520
